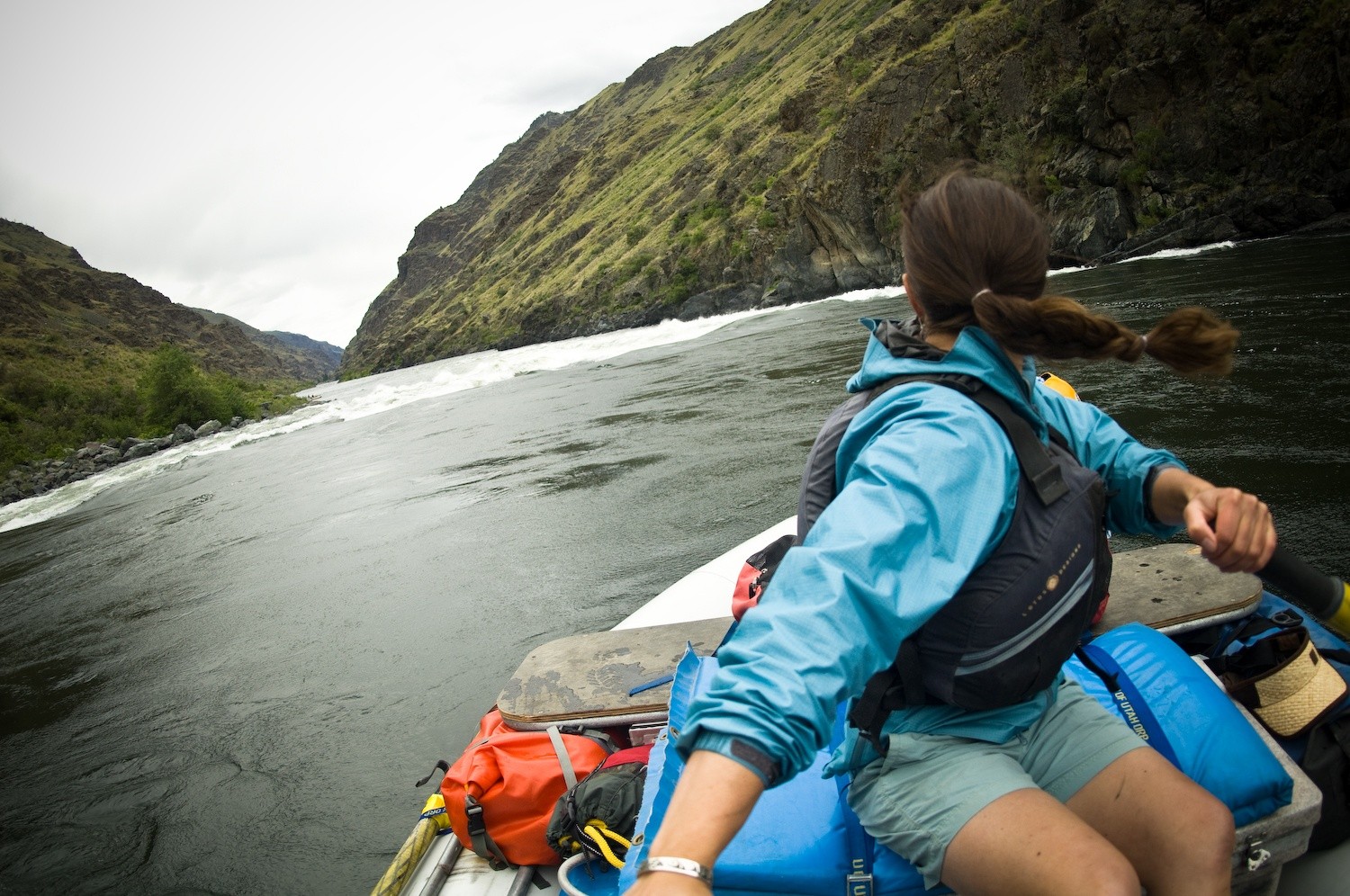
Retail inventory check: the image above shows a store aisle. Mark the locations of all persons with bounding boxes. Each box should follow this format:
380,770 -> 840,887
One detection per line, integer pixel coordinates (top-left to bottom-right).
618,170 -> 1278,896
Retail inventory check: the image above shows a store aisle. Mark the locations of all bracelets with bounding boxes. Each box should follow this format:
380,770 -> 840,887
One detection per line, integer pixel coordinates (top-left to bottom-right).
638,857 -> 712,890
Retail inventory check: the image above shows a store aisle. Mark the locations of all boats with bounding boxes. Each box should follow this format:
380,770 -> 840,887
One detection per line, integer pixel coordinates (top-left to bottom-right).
371,517 -> 1349,895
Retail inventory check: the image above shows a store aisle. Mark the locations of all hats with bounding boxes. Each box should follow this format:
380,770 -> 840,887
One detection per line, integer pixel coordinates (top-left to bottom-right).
1224,627 -> 1347,737
